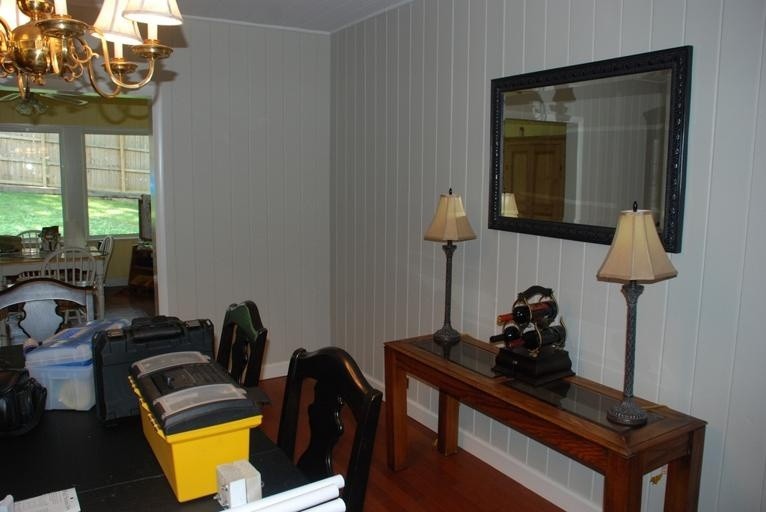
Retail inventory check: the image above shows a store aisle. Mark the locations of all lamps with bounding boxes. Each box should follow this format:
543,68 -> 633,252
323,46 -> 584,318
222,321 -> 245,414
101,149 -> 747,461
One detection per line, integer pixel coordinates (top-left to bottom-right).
0,0 -> 185,117
424,187 -> 479,346
595,201 -> 678,427
501,192 -> 519,218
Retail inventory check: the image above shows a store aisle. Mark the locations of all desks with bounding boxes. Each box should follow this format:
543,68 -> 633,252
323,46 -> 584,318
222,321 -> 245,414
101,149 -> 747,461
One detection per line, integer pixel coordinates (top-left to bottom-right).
384,335 -> 707,512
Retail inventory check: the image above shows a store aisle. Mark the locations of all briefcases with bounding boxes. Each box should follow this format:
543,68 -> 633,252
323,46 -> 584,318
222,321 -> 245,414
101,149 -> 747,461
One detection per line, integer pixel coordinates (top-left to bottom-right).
91,315 -> 215,422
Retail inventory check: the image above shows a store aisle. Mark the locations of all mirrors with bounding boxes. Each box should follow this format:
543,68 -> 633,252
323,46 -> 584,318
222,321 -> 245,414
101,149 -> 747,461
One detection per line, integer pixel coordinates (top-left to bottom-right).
487,45 -> 693,255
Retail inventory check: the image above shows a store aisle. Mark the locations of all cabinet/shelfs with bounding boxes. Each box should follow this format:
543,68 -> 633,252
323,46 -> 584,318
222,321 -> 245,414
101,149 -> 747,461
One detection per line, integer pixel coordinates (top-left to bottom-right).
502,137 -> 564,221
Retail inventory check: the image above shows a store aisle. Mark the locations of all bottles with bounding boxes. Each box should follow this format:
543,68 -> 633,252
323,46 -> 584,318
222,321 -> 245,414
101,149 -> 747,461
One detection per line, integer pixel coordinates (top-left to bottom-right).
488,302 -> 564,349
